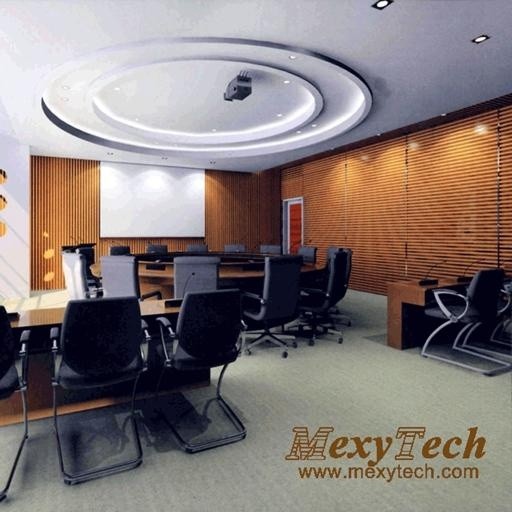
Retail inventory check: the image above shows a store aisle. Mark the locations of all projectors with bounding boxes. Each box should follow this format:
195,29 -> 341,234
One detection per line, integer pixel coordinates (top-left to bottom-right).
224,75 -> 253,101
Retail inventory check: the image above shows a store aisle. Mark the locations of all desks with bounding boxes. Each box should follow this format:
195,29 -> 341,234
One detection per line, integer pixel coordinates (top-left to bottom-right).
386,278 -> 470,350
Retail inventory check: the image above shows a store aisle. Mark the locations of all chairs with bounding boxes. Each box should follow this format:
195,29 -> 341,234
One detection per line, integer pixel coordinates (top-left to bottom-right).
0,244 -> 353,502
421,268 -> 511,376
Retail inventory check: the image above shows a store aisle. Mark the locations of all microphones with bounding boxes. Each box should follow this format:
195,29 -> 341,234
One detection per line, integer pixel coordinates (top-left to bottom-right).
457,258 -> 485,282
419,260 -> 447,285
164,272 -> 195,307
